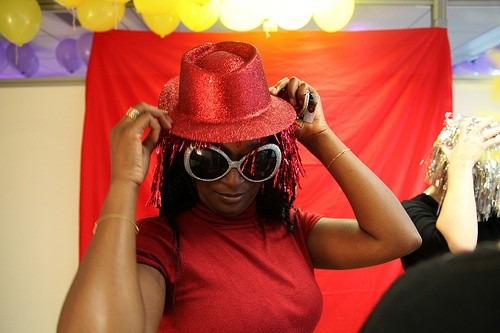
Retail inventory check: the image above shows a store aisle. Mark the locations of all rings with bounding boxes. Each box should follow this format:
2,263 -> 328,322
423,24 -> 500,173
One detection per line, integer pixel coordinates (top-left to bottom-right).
125,107 -> 140,120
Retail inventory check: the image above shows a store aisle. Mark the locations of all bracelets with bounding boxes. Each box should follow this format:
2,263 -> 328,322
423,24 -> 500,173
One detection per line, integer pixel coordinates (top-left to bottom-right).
327,148 -> 350,169
91,215 -> 140,234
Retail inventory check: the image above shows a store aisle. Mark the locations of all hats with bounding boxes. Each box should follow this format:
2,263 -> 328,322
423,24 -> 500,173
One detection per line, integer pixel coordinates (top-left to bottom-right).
156,42 -> 299,142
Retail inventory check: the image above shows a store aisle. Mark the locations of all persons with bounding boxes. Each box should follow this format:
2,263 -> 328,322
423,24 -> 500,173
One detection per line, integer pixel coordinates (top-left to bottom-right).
56,41 -> 423,333
399,112 -> 500,273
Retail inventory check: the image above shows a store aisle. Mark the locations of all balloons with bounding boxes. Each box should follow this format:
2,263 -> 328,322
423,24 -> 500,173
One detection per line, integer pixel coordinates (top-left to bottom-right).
55,39 -> 83,74
0,39 -> 9,74
262,19 -> 279,33
141,0 -> 180,38
77,0 -> 125,32
23,55 -> 39,78
55,0 -> 78,10
133,0 -> 142,13
268,0 -> 312,30
179,0 -> 220,32
219,0 -> 268,32
78,32 -> 95,66
6,44 -> 33,73
0,0 -> 42,47
312,0 -> 355,32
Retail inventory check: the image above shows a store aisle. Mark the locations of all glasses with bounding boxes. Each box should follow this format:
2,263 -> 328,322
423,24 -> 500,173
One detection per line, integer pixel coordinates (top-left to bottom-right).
182,142 -> 285,183
